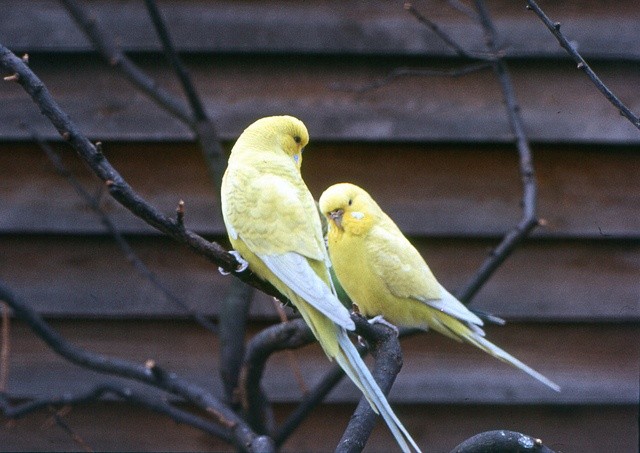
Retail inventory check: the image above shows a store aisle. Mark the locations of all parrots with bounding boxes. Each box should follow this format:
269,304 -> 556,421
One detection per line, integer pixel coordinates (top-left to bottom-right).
217,114 -> 423,452
318,182 -> 562,391
